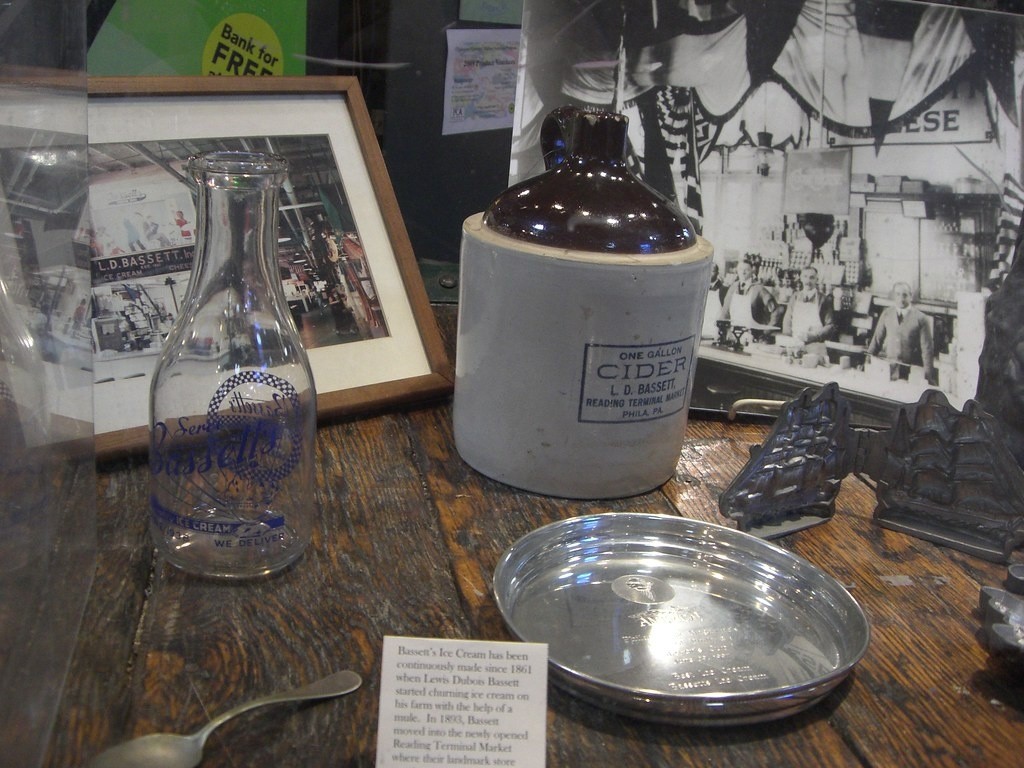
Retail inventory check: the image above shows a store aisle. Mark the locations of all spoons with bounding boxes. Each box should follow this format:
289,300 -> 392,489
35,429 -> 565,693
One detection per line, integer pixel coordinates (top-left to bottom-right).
84,670 -> 362,768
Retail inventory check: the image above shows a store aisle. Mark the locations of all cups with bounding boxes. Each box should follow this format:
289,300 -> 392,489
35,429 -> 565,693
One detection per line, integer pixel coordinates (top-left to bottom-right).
840,356 -> 850,368
803,353 -> 819,366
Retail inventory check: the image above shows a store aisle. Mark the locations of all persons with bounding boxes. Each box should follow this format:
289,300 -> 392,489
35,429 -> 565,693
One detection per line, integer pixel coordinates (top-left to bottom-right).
701,263 -> 724,339
123,207 -> 192,254
782,267 -> 838,355
720,260 -> 779,342
866,282 -> 935,380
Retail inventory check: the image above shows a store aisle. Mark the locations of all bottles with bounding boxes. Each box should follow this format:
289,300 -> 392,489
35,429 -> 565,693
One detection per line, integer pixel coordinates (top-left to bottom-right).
453,107 -> 715,498
144,150 -> 316,581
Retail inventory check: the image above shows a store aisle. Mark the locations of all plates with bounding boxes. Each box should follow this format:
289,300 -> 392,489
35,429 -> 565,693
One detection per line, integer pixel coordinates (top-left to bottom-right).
492,511 -> 870,726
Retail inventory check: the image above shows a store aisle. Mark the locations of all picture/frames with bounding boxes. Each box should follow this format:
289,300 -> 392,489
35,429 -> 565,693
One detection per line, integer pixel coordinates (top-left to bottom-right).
604,0 -> 1024,434
0,62 -> 450,476
